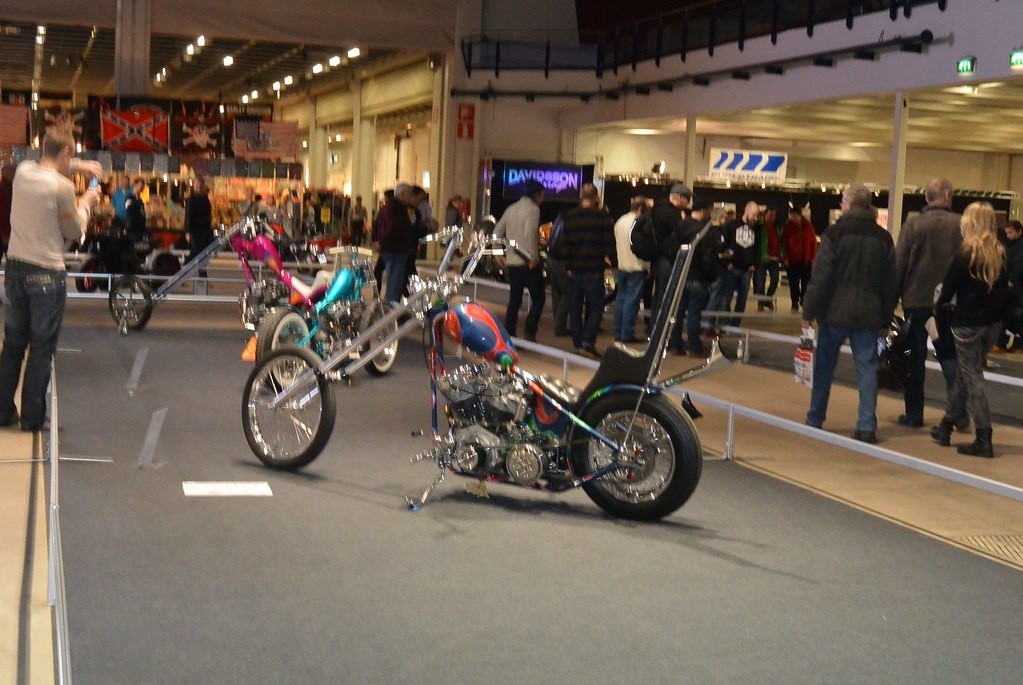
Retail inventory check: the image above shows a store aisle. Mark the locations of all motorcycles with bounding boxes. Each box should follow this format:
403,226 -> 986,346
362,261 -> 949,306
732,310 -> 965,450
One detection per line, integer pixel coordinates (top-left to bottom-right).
254,243 -> 401,395
243,203 -> 747,526
107,202 -> 335,361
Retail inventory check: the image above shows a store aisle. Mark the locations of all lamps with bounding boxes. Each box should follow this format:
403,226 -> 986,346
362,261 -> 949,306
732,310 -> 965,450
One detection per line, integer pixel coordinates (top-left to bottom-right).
763,64 -> 782,75
635,86 -> 649,94
812,56 -> 832,69
657,82 -> 672,91
854,48 -> 874,60
693,76 -> 709,85
732,70 -> 750,80
898,42 -> 922,53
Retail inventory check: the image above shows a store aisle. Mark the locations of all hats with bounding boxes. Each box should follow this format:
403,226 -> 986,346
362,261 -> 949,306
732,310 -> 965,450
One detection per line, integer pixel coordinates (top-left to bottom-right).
670,184 -> 694,197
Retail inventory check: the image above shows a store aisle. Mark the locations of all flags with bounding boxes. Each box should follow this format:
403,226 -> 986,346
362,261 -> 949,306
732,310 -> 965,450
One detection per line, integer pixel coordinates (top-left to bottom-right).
234,119 -> 298,158
29,108 -> 86,143
100,109 -> 168,153
171,117 -> 222,152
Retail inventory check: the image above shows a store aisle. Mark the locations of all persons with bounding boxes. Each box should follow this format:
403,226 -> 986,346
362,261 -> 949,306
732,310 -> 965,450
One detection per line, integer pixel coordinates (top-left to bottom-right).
248,189 -> 323,236
113,176 -> 147,238
931,201 -> 1012,458
444,194 -> 463,269
371,183 -> 431,304
895,179 -> 970,432
802,185 -> 898,442
493,182 -> 545,343
351,196 -> 368,246
0,130 -> 103,431
546,184 -> 817,357
185,177 -> 212,278
993,221 -> 1023,350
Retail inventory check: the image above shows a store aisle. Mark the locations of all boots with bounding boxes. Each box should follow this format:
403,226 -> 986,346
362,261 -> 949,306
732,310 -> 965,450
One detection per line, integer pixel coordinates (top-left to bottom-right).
957,428 -> 993,457
931,417 -> 954,446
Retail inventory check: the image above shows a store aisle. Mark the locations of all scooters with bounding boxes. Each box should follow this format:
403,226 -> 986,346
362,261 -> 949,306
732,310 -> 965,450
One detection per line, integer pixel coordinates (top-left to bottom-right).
75,226 -> 182,297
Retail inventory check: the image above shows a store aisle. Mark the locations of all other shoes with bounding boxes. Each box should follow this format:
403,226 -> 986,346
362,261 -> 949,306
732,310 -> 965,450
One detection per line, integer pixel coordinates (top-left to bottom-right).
758,305 -> 764,311
578,344 -> 602,361
899,415 -> 922,427
3,410 -> 18,423
523,333 -> 538,344
556,329 -> 572,337
671,348 -> 685,356
614,337 -> 622,343
22,420 -> 63,432
622,337 -> 644,343
851,430 -> 876,441
790,305 -> 799,312
766,301 -> 774,311
685,347 -> 710,358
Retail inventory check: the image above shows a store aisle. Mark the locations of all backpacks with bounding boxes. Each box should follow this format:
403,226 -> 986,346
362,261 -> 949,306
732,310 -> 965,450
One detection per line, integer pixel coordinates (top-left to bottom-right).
630,202 -> 661,262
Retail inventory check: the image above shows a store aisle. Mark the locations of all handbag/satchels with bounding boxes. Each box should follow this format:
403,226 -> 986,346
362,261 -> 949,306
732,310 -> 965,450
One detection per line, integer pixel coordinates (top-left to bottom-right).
794,326 -> 815,389
